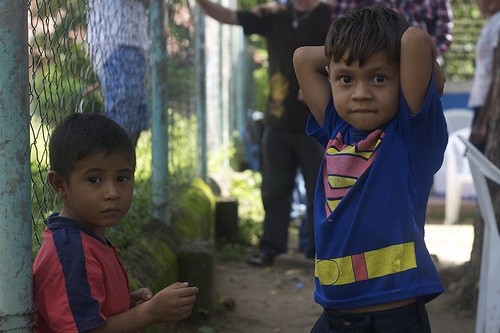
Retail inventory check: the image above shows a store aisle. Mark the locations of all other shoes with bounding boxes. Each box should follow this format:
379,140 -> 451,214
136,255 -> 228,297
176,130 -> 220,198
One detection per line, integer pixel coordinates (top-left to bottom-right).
249,250 -> 271,267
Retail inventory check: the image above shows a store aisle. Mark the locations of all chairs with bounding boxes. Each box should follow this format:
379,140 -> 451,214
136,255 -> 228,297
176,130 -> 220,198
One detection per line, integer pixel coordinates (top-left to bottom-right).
456,134 -> 500,333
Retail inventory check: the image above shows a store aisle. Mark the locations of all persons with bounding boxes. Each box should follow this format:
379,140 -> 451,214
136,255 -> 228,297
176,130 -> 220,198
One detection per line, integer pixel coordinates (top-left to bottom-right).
88,0 -> 500,313
33,113 -> 198,333
294,5 -> 448,333
